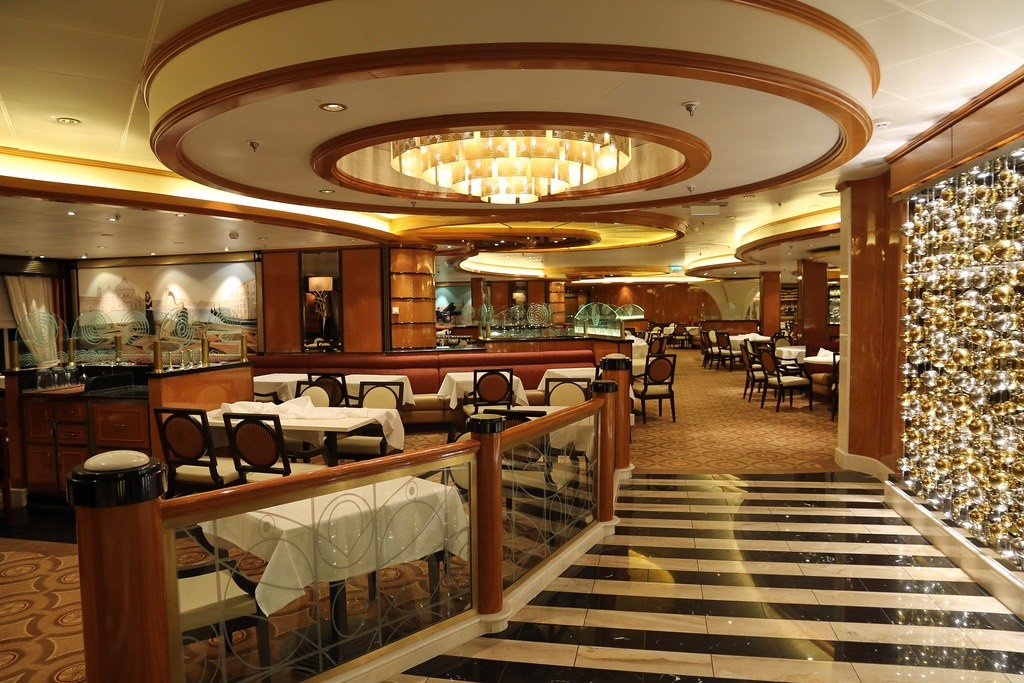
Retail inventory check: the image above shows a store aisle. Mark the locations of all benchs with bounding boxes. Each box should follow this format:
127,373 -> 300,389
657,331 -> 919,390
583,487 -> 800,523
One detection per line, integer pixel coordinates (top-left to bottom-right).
249,348 -> 597,430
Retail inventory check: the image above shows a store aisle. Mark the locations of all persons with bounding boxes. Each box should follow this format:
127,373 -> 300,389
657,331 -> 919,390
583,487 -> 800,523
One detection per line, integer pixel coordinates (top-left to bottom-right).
436,307 -> 443,321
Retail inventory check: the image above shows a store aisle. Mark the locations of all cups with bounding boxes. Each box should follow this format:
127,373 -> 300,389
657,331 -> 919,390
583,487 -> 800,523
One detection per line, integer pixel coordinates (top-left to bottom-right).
37,366 -> 81,389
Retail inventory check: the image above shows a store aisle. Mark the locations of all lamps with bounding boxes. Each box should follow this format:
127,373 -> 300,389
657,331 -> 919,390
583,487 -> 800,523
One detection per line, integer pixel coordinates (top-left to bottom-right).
308,276 -> 334,339
391,130 -> 631,205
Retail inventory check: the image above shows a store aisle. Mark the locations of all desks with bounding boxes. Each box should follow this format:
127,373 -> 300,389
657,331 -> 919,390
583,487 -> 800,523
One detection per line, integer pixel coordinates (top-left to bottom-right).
632,342 -> 649,359
686,326 -> 701,336
252,372 -> 321,402
189,401 -> 405,467
336,373 -> 417,406
198,475 -> 469,675
775,346 -> 806,366
536,367 -> 635,401
727,336 -> 771,357
632,359 -> 651,383
511,406 -> 595,464
802,356 -> 840,411
437,372 -> 529,410
664,327 -> 688,339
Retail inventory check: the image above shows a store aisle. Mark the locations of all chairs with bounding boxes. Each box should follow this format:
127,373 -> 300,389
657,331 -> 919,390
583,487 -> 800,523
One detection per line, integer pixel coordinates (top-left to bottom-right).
153,324 -> 840,683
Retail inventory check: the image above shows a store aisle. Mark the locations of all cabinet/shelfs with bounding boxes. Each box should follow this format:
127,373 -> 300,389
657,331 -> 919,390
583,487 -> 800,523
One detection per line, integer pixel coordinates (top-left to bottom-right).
21,397 -> 90,496
87,397 -> 153,458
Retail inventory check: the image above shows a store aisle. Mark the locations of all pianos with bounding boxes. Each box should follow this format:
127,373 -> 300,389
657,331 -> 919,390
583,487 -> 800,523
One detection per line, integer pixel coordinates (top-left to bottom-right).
441,302 -> 463,322
